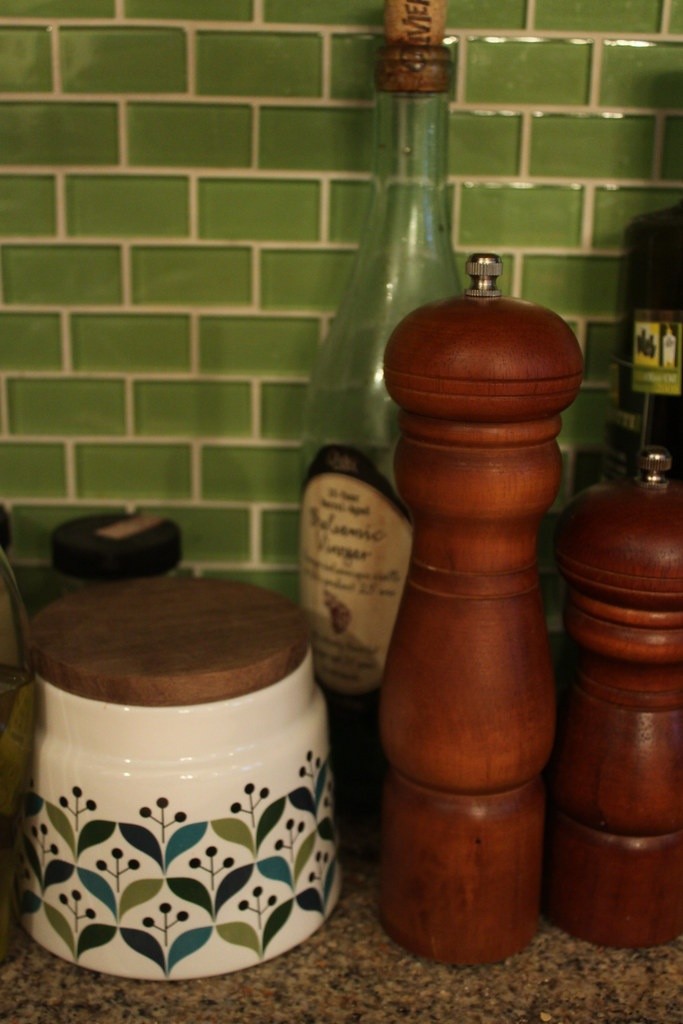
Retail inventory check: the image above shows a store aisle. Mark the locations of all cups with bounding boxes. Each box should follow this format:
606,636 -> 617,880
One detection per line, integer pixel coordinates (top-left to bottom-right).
10,578 -> 344,983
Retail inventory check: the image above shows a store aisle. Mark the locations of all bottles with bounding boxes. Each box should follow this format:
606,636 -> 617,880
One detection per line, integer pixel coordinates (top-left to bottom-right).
50,513 -> 197,598
385,250 -> 582,960
546,445 -> 682,947
296,0 -> 463,834
